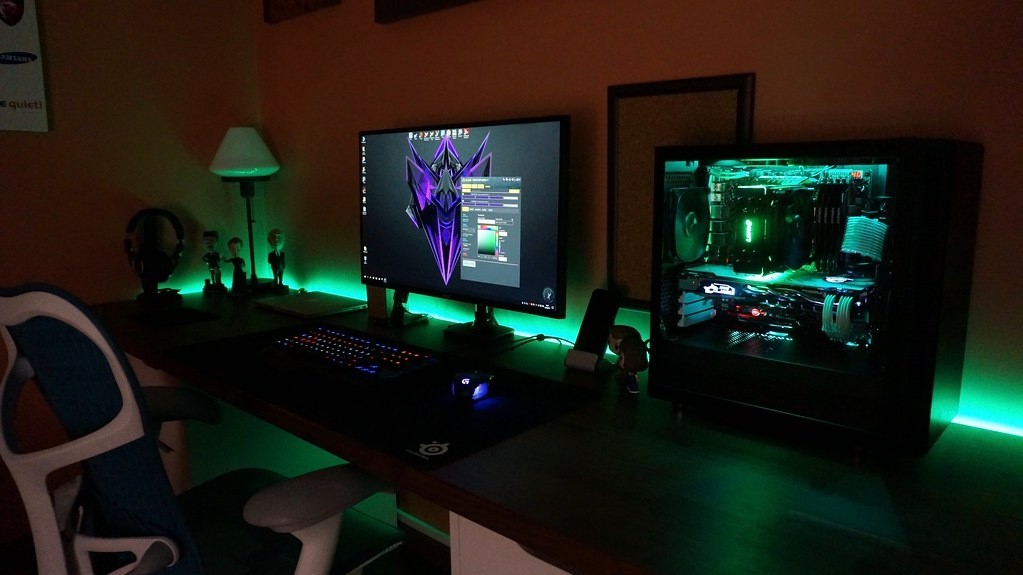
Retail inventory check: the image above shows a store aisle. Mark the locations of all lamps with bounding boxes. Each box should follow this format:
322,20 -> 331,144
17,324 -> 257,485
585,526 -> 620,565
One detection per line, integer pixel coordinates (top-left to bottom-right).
208,127 -> 281,295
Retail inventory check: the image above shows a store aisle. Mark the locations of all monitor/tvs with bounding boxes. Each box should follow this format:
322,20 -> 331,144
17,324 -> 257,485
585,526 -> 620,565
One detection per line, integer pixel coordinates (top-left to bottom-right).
649,137 -> 937,459
356,114 -> 572,346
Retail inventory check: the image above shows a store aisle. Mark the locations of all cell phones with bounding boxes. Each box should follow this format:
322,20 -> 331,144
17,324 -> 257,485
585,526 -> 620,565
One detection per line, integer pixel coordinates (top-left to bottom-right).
572,288 -> 619,360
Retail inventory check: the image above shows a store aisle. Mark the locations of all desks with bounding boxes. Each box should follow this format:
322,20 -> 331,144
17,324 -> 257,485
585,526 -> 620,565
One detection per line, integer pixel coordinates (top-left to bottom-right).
85,283 -> 1023,575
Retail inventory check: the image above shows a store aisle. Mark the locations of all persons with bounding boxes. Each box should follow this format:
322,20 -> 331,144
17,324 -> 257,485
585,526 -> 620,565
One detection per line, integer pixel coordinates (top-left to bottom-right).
223,237 -> 247,287
267,229 -> 287,286
609,326 -> 649,405
203,231 -> 221,288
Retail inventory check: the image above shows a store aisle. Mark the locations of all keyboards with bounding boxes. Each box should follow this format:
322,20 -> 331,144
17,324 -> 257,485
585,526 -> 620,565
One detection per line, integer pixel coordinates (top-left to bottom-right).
275,323 -> 442,390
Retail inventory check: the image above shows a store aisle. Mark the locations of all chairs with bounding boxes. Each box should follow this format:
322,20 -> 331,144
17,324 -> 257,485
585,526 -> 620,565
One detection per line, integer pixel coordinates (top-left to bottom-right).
0,282 -> 407,575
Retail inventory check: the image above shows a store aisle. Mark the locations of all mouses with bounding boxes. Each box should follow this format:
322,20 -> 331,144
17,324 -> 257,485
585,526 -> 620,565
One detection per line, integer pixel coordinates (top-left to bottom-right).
451,369 -> 496,403
